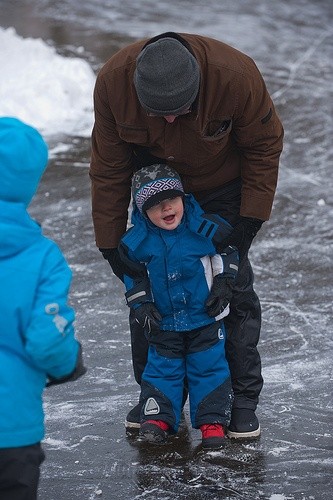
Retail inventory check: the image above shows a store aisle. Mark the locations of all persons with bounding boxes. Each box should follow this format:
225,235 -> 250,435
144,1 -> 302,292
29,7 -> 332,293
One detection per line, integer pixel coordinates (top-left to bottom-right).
0,116 -> 87,500
90,33 -> 287,438
121,163 -> 241,449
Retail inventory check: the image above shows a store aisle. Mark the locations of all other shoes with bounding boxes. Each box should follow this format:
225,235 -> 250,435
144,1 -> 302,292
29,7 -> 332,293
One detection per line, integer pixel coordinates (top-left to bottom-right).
227,408 -> 261,438
139,420 -> 169,443
200,424 -> 226,450
125,403 -> 184,429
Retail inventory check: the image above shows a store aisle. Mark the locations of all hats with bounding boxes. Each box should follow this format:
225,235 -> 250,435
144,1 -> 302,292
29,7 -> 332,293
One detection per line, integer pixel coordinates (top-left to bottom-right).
132,163 -> 185,219
134,36 -> 201,111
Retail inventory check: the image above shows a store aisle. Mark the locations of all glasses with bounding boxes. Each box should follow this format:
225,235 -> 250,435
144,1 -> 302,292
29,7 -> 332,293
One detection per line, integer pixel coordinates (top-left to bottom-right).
146,107 -> 193,117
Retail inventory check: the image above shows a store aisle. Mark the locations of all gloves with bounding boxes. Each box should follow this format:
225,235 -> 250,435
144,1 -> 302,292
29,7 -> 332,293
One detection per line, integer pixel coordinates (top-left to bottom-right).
216,218 -> 265,261
97,247 -> 140,283
207,275 -> 237,318
132,303 -> 162,337
